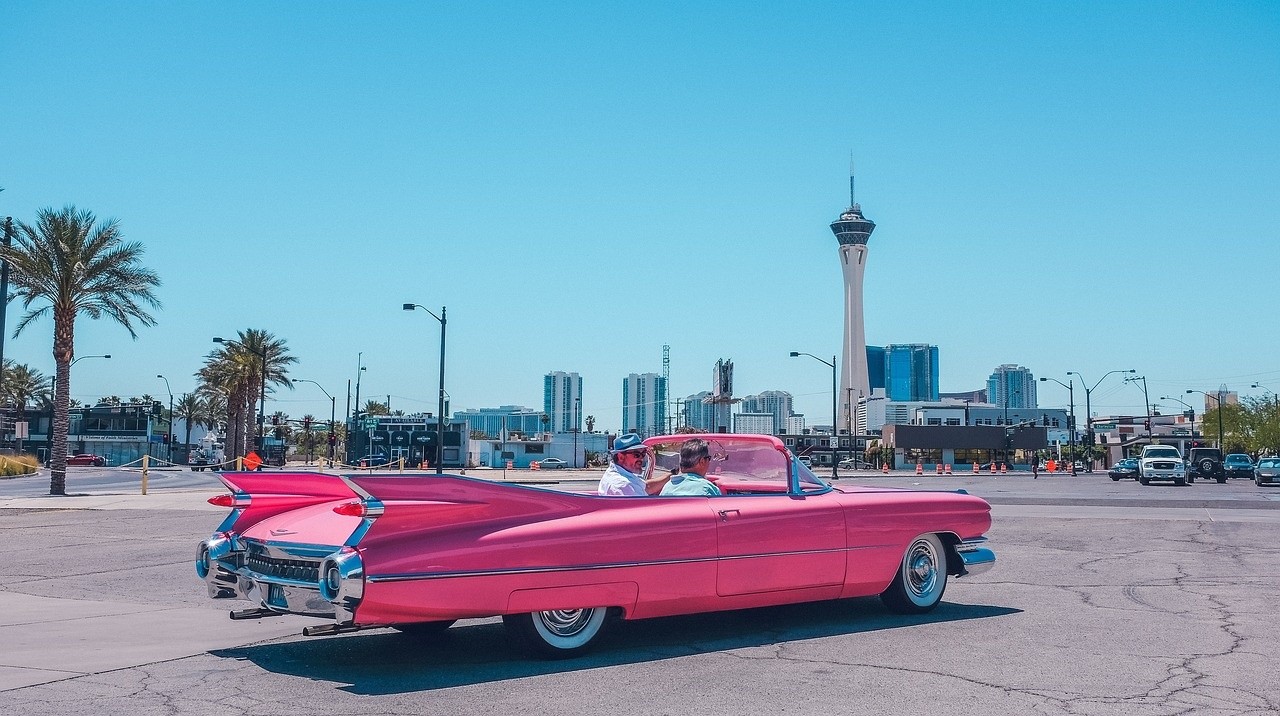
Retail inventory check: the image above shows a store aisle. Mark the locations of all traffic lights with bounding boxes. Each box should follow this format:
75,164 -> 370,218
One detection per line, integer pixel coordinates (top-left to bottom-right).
1187,440 -> 1191,449
162,433 -> 169,444
276,428 -> 280,440
271,414 -> 278,425
154,401 -> 161,413
304,417 -> 310,428
158,413 -> 163,424
329,435 -> 335,446
1193,441 -> 1198,447
1143,419 -> 1150,430
150,410 -> 154,420
173,433 -> 178,443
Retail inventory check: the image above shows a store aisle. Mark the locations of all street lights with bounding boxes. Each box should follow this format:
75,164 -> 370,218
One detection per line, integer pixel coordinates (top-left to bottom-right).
1185,388 -> 1223,460
1161,395 -> 1195,448
291,378 -> 335,467
1003,388 -> 1020,467
789,349 -> 839,480
1039,375 -> 1078,478
213,335 -> 268,471
1067,367 -> 1137,472
573,396 -> 581,469
402,301 -> 448,474
42,354 -> 112,466
1251,383 -> 1278,423
157,373 -> 174,467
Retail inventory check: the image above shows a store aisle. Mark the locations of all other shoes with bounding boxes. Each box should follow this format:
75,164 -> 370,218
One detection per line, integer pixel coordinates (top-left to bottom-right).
1034,475 -> 1037,479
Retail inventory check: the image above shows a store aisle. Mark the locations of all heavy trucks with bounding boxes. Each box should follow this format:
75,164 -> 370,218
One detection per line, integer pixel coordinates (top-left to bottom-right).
189,432 -> 227,472
252,436 -> 290,468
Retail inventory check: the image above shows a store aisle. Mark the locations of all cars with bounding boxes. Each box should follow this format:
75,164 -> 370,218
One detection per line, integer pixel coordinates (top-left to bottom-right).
978,460 -> 1014,471
61,453 -> 104,467
528,458 -> 569,469
838,459 -> 875,470
1221,453 -> 1256,479
43,455 -> 75,466
1253,458 -> 1280,486
1108,457 -> 1140,481
1070,462 -> 1083,471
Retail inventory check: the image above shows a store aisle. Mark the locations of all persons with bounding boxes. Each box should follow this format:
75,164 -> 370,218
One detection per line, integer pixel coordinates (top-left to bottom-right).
658,439 -> 722,495
598,433 -> 679,497
1032,451 -> 1039,479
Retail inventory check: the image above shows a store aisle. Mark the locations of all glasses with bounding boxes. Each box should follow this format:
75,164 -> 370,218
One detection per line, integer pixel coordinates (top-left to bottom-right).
625,450 -> 646,458
705,455 -> 710,460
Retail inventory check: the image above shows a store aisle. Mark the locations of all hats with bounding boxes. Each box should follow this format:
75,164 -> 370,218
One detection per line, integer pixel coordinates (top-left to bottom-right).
610,433 -> 647,455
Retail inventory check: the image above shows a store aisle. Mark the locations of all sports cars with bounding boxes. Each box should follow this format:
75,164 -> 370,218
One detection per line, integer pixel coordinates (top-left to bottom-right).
187,431 -> 1002,663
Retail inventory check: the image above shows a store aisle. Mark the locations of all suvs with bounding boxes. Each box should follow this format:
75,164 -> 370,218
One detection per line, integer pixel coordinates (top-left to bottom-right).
352,454 -> 389,469
1135,444 -> 1188,485
798,454 -> 812,471
1186,448 -> 1226,484
